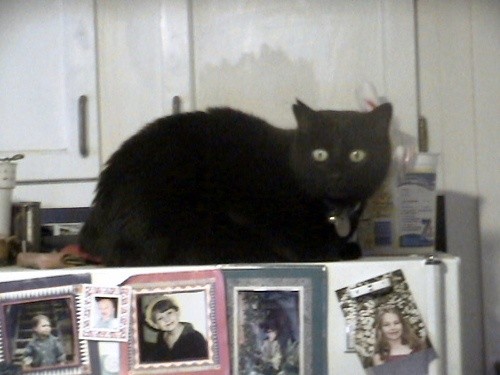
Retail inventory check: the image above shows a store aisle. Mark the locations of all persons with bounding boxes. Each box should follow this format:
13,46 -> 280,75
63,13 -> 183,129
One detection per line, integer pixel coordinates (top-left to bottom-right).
23,315 -> 66,367
95,298 -> 117,328
373,304 -> 424,365
144,296 -> 207,359
255,307 -> 298,375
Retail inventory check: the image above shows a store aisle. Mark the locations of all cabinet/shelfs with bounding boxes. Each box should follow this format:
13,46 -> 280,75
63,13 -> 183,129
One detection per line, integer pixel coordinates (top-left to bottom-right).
0,0 -> 101,182
96,1 -> 418,175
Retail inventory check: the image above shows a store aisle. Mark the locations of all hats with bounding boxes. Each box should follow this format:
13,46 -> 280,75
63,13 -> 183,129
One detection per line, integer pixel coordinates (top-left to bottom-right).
142,295 -> 180,330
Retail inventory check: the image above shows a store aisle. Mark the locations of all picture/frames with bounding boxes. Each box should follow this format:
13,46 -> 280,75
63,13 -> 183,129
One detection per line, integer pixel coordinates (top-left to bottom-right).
219,266 -> 329,375
119,271 -> 229,374
0,273 -> 102,374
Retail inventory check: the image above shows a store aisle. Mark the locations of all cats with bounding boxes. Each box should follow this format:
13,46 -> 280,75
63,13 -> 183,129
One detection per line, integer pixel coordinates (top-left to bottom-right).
78,103 -> 392,266
